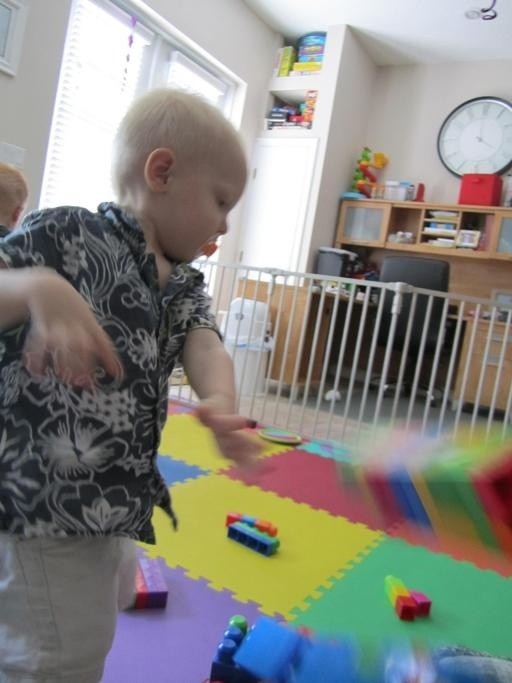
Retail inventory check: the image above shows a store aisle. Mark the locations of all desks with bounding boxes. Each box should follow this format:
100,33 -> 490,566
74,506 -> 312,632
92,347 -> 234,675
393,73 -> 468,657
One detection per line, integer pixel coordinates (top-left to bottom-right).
324,193 -> 510,417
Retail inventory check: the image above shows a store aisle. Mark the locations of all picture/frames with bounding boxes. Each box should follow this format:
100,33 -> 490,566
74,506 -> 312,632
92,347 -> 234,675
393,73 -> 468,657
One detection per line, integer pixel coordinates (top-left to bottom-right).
1,0 -> 34,82
486,287 -> 510,316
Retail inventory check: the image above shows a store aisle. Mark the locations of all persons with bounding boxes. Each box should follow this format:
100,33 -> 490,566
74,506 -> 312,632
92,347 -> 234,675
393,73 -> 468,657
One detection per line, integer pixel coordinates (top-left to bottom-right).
2,90 -> 265,682
1,162 -> 27,236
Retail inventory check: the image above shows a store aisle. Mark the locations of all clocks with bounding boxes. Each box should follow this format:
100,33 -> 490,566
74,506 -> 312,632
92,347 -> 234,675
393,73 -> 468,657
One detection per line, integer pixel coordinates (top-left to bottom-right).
434,93 -> 511,180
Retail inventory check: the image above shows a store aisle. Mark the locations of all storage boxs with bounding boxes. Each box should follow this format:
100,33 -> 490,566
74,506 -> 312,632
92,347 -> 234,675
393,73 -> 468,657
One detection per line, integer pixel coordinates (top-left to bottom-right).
456,171 -> 503,207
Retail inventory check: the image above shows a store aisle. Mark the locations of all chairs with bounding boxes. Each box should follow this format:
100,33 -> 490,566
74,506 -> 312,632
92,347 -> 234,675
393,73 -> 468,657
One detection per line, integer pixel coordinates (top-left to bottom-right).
359,254 -> 462,406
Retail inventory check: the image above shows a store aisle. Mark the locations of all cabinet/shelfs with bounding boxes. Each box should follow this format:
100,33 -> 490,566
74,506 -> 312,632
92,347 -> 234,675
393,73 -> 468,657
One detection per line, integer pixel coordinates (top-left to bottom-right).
228,279 -> 336,402
233,75 -> 320,283
